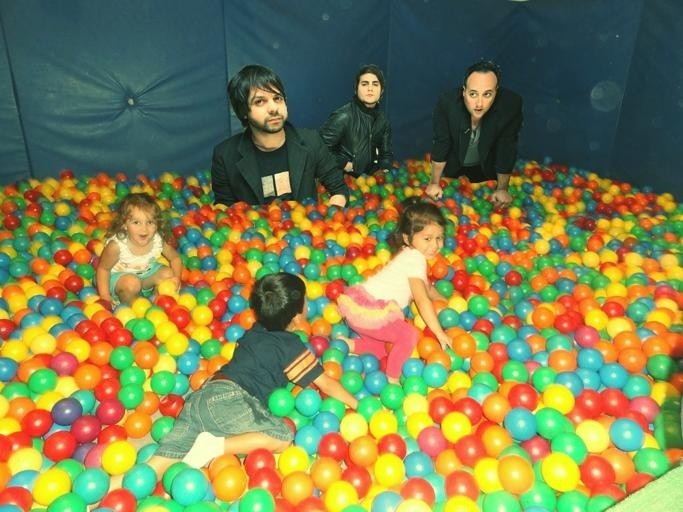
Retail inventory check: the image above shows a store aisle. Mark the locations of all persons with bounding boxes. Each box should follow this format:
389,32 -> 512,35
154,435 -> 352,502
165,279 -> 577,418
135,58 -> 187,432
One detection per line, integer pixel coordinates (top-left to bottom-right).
338,196 -> 454,384
210,64 -> 350,209
319,62 -> 393,175
85,273 -> 358,511
425,61 -> 523,210
96,191 -> 181,307
